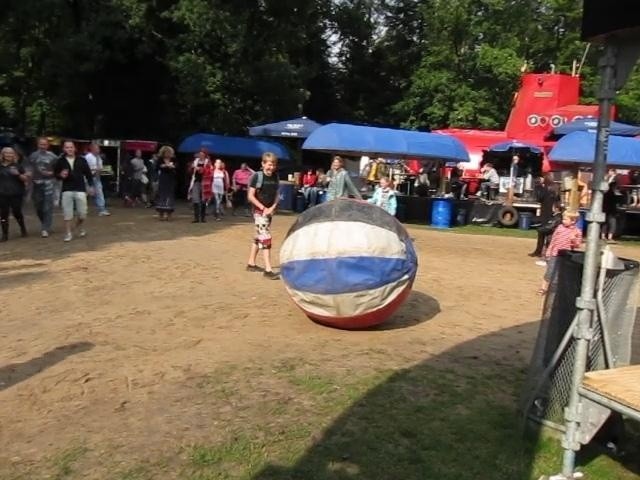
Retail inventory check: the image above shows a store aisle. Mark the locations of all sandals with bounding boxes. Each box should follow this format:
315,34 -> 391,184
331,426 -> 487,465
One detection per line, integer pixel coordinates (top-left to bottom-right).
537,289 -> 545,295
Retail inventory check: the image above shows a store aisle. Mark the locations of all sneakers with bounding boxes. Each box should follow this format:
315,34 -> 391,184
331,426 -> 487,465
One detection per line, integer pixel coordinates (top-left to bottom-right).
41,230 -> 48,237
248,265 -> 280,276
64,229 -> 86,241
99,211 -> 110,216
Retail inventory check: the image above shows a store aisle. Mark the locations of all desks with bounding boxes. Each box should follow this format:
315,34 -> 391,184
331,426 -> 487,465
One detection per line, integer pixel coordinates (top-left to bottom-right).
619,185 -> 640,209
462,176 -> 484,179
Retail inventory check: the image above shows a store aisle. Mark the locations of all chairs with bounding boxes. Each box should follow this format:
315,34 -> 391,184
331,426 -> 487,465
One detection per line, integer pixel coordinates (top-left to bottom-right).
392,173 -> 416,196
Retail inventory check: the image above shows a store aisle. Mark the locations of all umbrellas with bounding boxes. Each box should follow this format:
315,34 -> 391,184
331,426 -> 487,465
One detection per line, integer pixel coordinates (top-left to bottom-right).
248,116 -> 323,139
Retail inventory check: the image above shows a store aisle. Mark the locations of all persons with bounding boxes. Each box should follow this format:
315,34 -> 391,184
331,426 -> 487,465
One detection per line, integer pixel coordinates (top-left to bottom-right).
1,138 -> 109,242
534,207 -> 583,297
404,153 -> 620,258
248,151 -> 283,277
302,156 -> 397,215
128,150 -> 257,221
